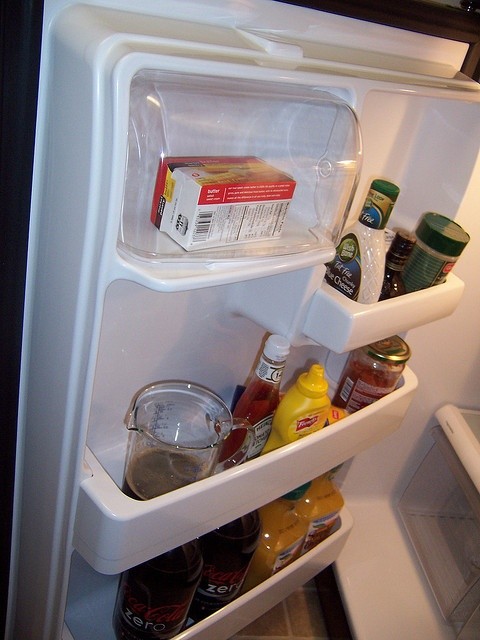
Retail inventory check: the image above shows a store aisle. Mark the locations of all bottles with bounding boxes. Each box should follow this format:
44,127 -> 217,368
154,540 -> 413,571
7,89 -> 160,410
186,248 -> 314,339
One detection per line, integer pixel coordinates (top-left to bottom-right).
401,212 -> 471,294
324,179 -> 401,304
218,334 -> 292,471
378,230 -> 417,302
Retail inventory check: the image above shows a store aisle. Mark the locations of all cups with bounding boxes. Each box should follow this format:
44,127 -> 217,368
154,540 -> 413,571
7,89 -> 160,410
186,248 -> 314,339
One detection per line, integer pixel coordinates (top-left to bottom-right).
122,380 -> 255,502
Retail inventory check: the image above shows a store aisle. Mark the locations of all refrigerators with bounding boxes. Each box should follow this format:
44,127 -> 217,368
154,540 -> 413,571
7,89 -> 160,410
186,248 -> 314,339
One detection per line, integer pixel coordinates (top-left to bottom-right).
0,0 -> 480,640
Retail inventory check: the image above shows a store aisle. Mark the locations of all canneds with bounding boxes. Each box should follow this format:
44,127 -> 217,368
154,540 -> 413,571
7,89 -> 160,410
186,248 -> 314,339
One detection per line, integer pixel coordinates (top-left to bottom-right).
407,210 -> 472,296
